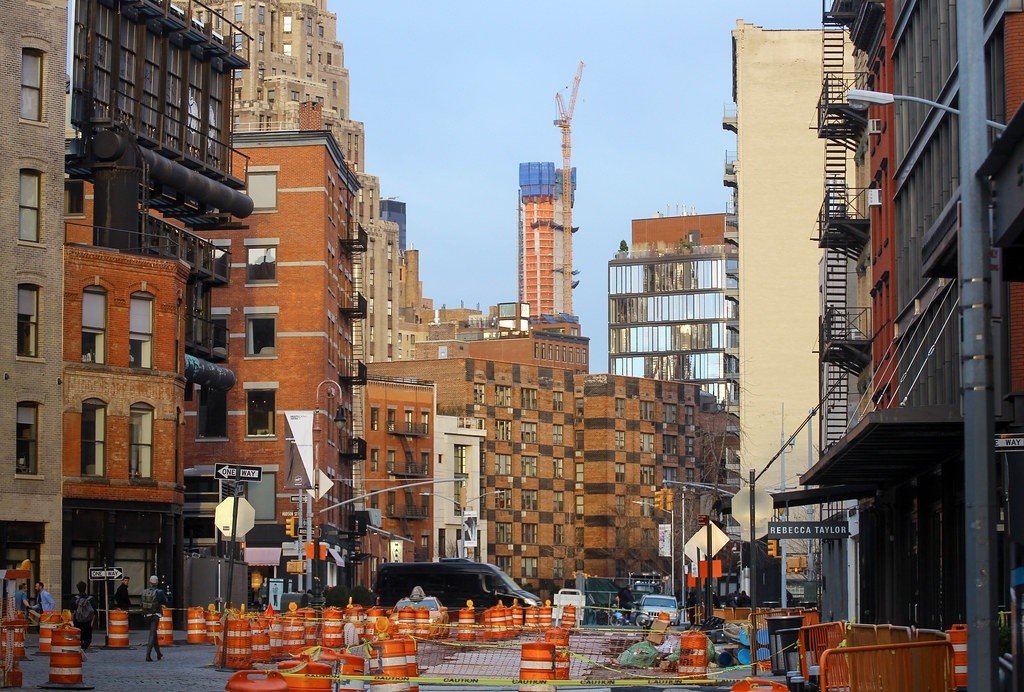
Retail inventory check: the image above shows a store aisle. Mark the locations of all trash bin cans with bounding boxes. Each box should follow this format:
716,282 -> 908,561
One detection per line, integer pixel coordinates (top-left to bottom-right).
765,615 -> 806,676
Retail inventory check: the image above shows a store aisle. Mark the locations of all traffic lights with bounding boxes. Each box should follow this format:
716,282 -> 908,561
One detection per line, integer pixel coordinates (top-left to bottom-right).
654,489 -> 664,511
285,518 -> 294,536
767,540 -> 777,558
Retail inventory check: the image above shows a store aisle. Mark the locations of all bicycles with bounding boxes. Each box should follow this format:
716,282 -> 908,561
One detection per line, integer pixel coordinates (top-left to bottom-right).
610,602 -> 651,627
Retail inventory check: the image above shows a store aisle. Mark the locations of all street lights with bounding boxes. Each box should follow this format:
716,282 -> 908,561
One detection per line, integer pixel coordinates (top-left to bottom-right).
418,491 -> 505,557
631,500 -> 674,595
310,379 -> 347,609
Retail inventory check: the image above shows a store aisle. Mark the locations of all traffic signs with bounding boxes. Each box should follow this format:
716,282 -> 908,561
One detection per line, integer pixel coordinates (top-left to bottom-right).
88,566 -> 123,581
213,462 -> 262,483
298,528 -> 321,537
289,495 -> 308,504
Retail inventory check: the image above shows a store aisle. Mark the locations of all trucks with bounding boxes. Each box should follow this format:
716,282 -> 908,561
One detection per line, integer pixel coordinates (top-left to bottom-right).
630,572 -> 671,600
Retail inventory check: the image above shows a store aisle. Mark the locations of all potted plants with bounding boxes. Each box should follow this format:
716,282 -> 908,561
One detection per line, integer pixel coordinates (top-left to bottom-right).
682,238 -> 693,255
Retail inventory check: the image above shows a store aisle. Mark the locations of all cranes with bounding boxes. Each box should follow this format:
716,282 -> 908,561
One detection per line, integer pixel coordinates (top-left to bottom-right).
552,60 -> 586,317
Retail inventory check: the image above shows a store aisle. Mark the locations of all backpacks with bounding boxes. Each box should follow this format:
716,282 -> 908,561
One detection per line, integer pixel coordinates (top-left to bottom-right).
142,589 -> 158,612
75,595 -> 94,622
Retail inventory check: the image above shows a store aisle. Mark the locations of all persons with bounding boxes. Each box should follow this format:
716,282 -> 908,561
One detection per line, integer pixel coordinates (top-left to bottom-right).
686,588 -> 751,626
28,582 -> 55,635
259,576 -> 268,612
114,576 -> 131,628
15,583 -> 30,617
273,584 -> 279,607
140,575 -> 167,661
67,581 -> 97,662
251,588 -> 261,607
300,589 -> 314,608
321,584 -> 332,608
620,584 -> 636,621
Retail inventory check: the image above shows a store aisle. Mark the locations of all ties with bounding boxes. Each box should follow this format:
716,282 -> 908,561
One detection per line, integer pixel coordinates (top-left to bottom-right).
38,594 -> 43,612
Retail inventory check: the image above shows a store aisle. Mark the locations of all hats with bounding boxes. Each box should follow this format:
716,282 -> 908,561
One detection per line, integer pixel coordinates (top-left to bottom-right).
150,575 -> 158,583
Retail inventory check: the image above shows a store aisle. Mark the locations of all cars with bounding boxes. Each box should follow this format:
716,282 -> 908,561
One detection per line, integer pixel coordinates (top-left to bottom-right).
633,594 -> 685,626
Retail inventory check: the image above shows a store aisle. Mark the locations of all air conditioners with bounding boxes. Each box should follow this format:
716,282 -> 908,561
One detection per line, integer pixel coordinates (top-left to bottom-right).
617,241 -> 630,260
867,188 -> 883,204
867,117 -> 882,135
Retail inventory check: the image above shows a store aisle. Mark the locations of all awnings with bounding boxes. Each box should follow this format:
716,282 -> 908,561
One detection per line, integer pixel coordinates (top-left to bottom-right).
326,548 -> 344,567
244,547 -> 281,566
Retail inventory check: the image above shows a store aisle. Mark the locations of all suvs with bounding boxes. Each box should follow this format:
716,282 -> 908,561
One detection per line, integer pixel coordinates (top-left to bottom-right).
371,557 -> 543,609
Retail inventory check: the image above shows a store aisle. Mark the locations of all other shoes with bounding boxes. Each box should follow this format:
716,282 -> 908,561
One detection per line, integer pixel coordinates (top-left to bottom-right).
157,652 -> 163,660
146,656 -> 153,661
